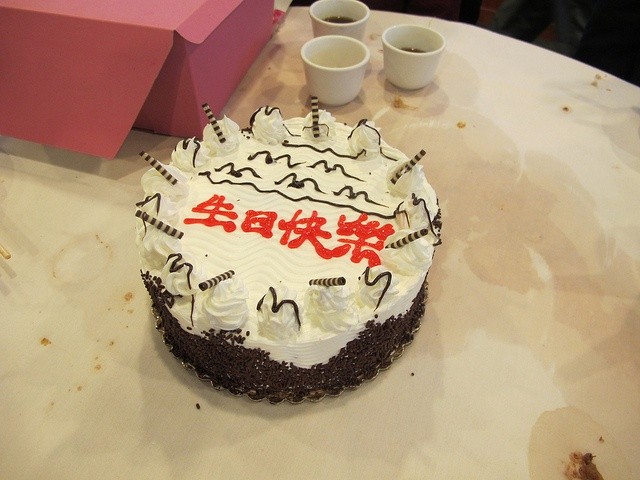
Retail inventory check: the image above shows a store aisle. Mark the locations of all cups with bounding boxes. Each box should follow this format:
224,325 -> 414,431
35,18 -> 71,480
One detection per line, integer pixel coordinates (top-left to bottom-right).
301,34 -> 370,106
309,0 -> 370,42
382,24 -> 445,90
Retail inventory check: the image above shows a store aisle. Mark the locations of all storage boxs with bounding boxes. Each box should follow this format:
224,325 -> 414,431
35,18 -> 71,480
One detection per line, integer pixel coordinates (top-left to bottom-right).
1,0 -> 293,159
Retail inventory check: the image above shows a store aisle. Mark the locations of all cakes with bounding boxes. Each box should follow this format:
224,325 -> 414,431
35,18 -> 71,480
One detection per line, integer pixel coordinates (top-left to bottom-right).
134,95 -> 442,406
